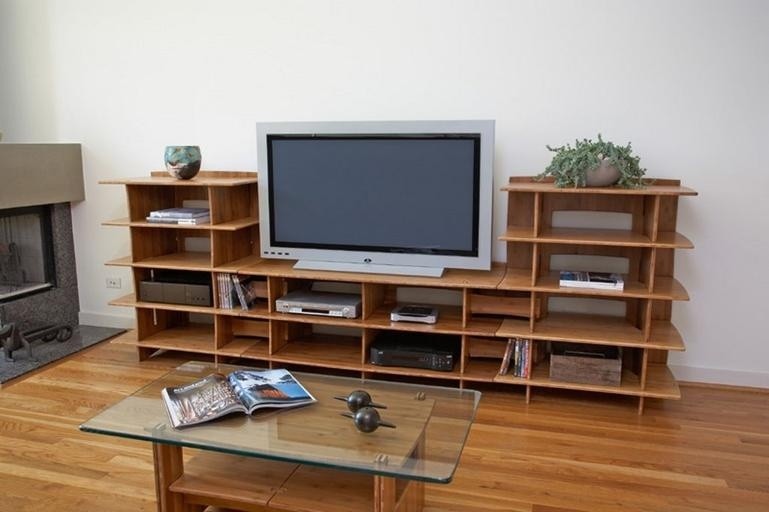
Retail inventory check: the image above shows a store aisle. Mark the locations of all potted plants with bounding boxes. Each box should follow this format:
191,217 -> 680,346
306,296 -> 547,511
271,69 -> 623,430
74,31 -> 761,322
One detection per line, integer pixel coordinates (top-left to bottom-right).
533,136 -> 660,192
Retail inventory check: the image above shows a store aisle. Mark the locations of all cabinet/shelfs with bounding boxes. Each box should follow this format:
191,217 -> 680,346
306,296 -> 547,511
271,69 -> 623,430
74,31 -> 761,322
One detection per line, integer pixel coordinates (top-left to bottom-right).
97,170 -> 699,415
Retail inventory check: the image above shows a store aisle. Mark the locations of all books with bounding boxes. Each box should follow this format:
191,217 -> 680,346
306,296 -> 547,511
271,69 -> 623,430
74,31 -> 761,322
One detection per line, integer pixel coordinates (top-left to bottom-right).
145,206 -> 210,225
161,367 -> 318,431
499,337 -> 528,378
216,272 -> 257,311
560,269 -> 624,291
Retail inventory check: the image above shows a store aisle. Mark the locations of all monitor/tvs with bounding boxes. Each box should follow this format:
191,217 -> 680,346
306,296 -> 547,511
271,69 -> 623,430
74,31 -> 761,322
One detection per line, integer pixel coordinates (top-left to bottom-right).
255,119 -> 496,278
